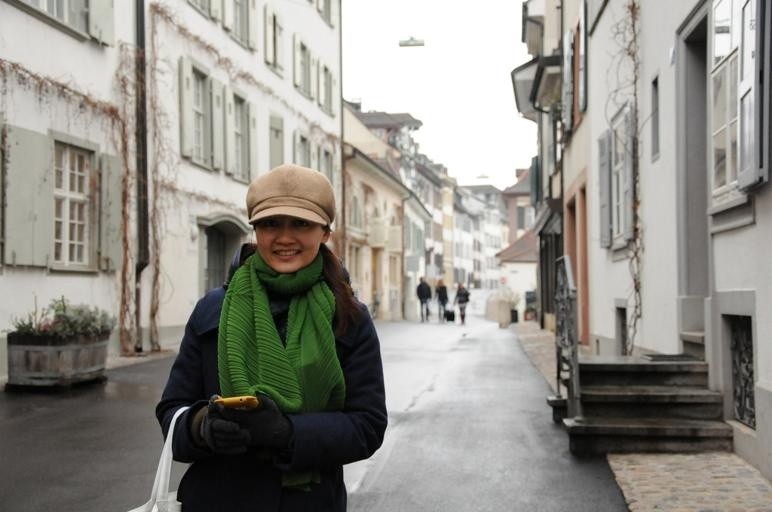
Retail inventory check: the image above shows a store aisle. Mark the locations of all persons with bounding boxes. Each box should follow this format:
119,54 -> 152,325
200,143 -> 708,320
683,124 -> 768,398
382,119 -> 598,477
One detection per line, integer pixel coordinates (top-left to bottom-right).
452,281 -> 471,324
433,278 -> 449,321
149,162 -> 389,512
416,276 -> 432,323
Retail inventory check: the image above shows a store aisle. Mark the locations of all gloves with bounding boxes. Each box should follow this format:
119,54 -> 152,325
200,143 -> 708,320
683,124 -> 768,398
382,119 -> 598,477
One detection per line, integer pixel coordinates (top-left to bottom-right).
191,393 -> 292,456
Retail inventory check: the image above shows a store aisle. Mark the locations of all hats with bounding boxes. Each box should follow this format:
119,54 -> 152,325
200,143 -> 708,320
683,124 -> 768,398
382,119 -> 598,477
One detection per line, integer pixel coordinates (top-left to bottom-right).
247,165 -> 336,226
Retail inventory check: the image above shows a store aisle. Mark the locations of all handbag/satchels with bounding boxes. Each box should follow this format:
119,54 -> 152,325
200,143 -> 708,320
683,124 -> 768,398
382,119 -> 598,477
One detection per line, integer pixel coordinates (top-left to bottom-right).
124,405 -> 182,512
447,311 -> 454,321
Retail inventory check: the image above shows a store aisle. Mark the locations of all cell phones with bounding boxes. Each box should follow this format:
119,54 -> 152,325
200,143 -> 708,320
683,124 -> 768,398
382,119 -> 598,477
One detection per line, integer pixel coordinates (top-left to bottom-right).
216,396 -> 258,410
